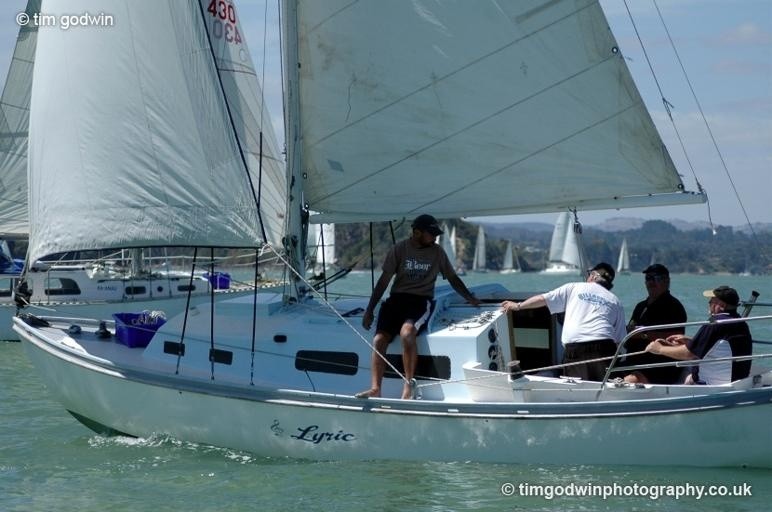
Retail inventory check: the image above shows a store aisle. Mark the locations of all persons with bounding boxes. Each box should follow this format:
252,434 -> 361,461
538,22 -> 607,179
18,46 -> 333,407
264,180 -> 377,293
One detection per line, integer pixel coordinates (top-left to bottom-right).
641,285 -> 755,388
611,263 -> 688,386
353,213 -> 483,408
498,261 -> 630,384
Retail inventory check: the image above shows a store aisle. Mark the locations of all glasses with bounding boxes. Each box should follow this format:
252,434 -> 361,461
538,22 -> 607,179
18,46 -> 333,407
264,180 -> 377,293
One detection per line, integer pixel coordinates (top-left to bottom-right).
645,274 -> 664,282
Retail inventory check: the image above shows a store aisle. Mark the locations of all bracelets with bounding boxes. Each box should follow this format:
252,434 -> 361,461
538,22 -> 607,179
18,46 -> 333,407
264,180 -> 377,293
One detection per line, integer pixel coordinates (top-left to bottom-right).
516,301 -> 522,310
464,292 -> 473,301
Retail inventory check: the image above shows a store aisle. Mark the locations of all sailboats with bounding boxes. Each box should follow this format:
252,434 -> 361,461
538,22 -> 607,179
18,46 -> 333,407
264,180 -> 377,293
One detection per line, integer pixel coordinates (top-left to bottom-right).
13,0 -> 772,472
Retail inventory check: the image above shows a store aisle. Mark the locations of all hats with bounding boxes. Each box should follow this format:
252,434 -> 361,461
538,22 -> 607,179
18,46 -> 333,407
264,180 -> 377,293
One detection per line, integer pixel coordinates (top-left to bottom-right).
410,215 -> 444,235
586,262 -> 615,284
703,285 -> 738,305
642,264 -> 668,275
